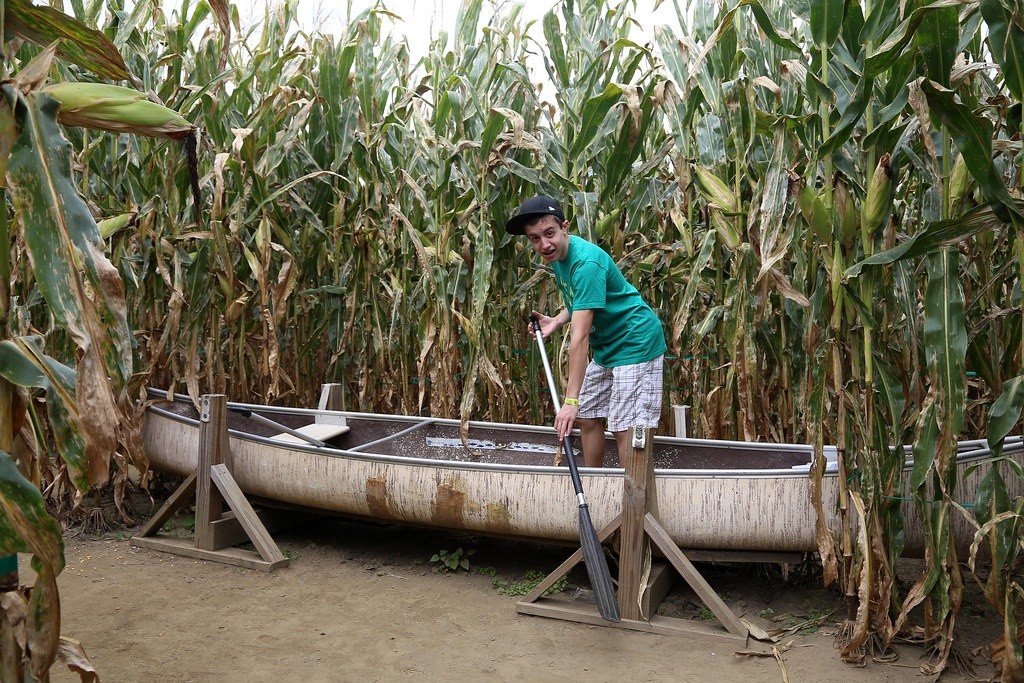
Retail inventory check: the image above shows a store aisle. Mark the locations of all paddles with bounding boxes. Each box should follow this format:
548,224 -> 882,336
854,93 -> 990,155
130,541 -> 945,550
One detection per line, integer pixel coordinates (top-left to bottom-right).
532,316 -> 624,623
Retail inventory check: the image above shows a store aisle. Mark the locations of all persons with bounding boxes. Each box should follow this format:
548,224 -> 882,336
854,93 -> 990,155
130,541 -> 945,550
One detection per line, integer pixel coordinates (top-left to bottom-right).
505,197 -> 668,467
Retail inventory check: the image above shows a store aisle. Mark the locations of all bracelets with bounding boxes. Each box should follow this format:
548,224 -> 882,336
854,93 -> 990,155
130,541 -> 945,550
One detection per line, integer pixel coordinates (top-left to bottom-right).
565,398 -> 579,406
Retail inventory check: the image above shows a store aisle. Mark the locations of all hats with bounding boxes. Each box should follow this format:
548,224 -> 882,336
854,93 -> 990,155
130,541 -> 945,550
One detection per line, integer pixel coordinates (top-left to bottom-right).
506,195 -> 565,235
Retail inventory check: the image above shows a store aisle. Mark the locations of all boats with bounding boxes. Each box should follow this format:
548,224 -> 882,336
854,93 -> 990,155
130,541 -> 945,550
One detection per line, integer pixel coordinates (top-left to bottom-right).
142,384 -> 1023,561
